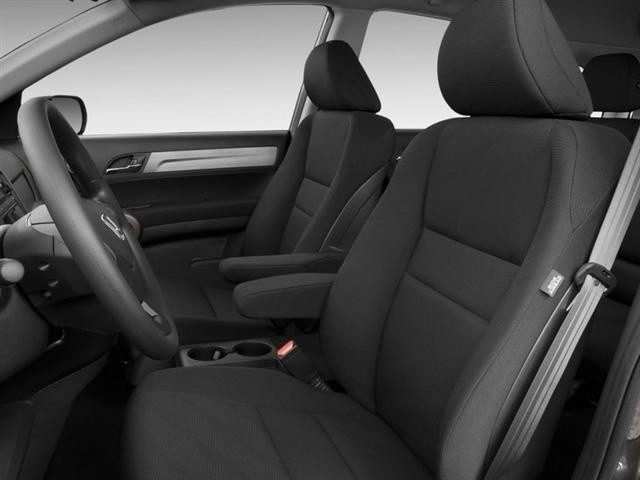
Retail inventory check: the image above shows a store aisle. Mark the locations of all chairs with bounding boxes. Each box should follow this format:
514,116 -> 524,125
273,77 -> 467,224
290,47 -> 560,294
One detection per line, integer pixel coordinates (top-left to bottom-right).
579,50 -> 639,144
116,38 -> 400,375
119,1 -> 633,480
